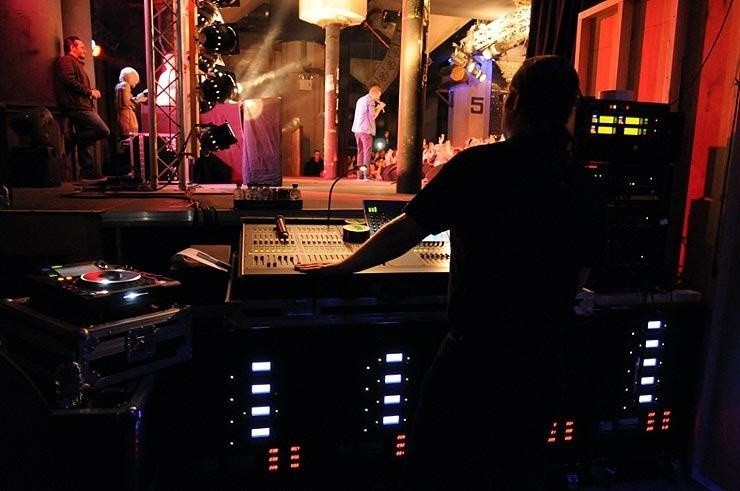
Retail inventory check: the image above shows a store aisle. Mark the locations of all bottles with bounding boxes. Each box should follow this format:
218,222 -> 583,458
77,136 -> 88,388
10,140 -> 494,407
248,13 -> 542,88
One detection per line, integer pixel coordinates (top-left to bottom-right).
232,181 -> 302,201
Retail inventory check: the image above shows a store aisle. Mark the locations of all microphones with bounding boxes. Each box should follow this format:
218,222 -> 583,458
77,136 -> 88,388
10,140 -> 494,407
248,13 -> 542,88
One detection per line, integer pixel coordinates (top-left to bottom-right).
377,100 -> 385,113
275,214 -> 289,240
328,165 -> 367,220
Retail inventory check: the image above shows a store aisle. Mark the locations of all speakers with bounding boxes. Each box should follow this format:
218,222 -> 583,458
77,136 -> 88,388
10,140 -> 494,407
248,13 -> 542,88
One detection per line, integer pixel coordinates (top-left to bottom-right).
365,11 -> 401,96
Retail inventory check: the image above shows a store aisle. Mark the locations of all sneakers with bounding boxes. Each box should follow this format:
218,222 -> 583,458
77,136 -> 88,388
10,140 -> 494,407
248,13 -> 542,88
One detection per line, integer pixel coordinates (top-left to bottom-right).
81,176 -> 108,183
357,176 -> 375,183
61,132 -> 74,164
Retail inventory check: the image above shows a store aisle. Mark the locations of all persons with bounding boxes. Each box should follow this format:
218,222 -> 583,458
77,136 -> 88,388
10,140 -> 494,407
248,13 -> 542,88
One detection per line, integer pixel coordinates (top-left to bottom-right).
303,128 -> 510,182
55,36 -> 111,180
294,54 -> 608,491
351,86 -> 387,180
114,66 -> 149,178
